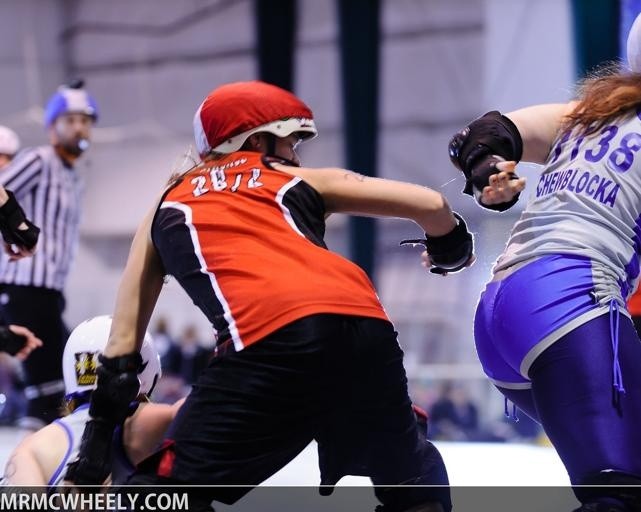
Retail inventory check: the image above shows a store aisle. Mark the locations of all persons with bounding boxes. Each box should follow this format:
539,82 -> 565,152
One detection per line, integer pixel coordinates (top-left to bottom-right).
57,81 -> 477,511
449,0 -> 641,512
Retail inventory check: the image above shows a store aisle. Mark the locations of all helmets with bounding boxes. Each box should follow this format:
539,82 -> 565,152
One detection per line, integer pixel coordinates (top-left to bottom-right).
61,316 -> 162,398
627,13 -> 641,72
44,83 -> 98,127
192,80 -> 318,159
